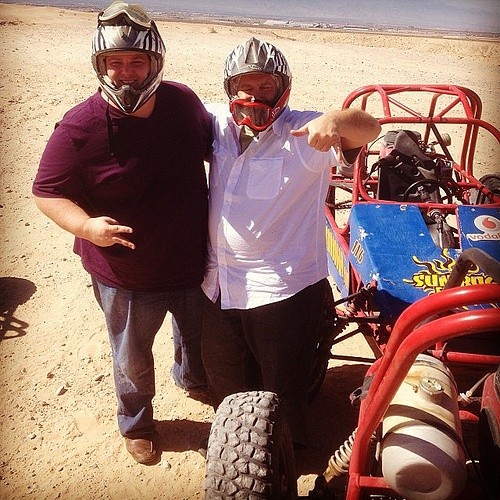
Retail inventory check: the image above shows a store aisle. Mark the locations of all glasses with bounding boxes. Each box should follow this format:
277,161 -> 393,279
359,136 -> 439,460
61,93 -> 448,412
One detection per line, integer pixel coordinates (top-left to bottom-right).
229,86 -> 291,131
97,1 -> 161,38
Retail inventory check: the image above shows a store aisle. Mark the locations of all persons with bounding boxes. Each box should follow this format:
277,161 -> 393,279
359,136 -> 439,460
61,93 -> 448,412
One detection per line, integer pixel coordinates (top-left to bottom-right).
197,36 -> 383,460
32,0 -> 214,463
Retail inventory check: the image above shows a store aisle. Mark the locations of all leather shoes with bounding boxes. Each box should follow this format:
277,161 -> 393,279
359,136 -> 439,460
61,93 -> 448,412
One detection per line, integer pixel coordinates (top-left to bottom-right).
123,435 -> 158,462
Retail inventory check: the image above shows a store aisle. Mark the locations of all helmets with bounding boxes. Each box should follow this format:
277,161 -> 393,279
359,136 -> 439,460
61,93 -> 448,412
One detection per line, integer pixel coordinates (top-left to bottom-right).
223,36 -> 292,108
91,19 -> 166,115
